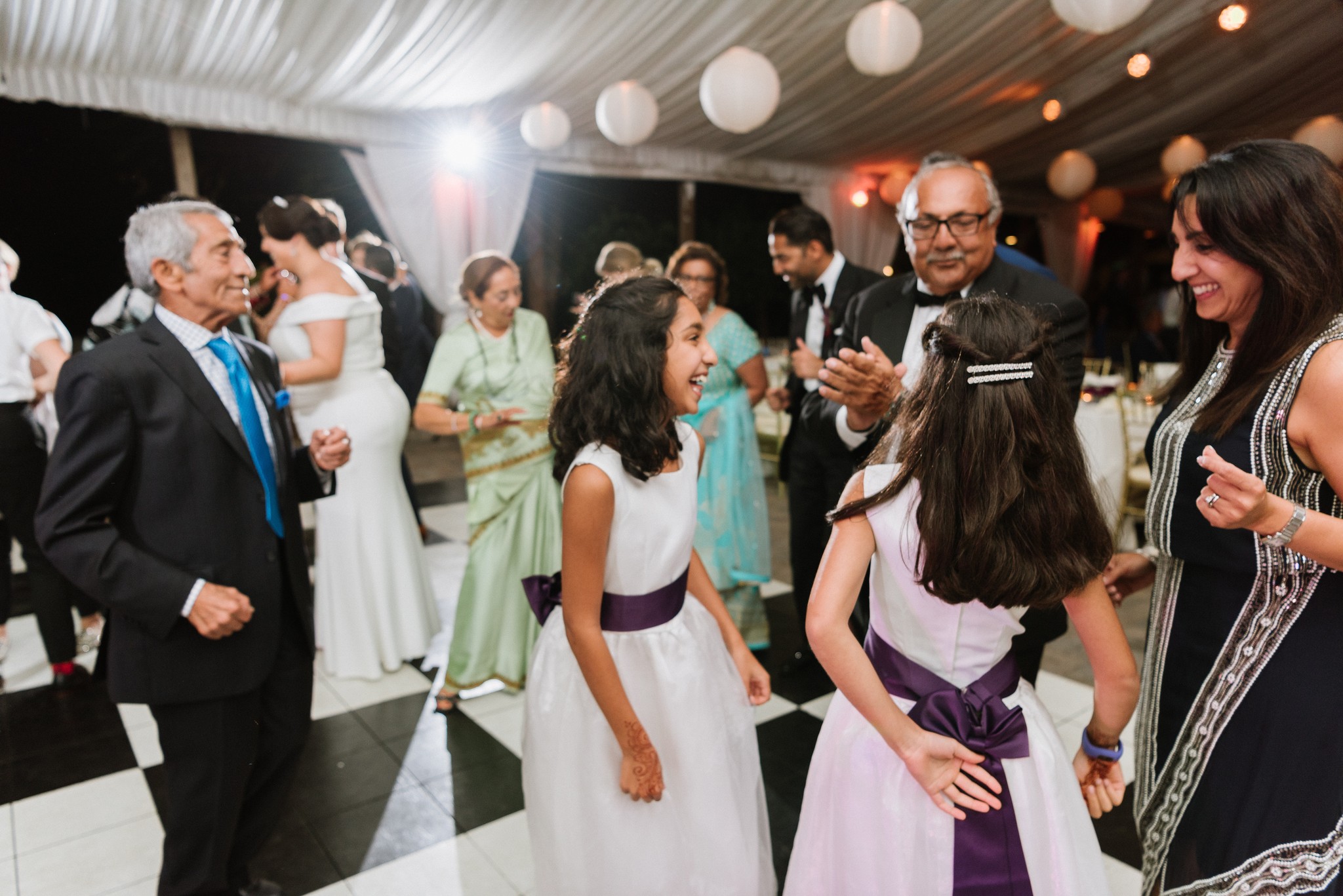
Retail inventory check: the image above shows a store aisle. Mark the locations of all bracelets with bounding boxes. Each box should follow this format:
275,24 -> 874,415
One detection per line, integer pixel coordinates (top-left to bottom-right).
451,411 -> 460,435
884,386 -> 909,424
475,413 -> 484,431
467,410 -> 480,436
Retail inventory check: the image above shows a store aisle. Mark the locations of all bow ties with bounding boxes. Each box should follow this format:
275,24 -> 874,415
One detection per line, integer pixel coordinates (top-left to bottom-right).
802,283 -> 827,306
913,288 -> 963,308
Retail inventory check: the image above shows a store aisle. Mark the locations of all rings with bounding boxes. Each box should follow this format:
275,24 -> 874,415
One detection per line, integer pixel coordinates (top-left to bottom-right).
1205,492 -> 1220,510
497,415 -> 502,420
341,437 -> 350,447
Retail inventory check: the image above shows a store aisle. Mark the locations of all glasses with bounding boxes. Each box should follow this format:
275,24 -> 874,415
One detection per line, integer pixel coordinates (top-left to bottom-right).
904,206 -> 992,241
676,273 -> 715,286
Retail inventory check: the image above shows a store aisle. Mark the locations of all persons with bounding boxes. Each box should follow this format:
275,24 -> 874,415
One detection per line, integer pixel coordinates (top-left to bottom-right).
920,148 -> 1066,282
798,158 -> 1090,709
0,238 -> 107,658
1094,134 -> 1343,896
513,269 -> 784,896
0,258 -> 88,699
594,241 -> 645,290
763,203 -> 888,680
86,185 -> 433,539
662,240 -> 777,667
256,195 -> 444,680
773,286 -> 1143,895
411,249 -> 564,716
30,200 -> 357,895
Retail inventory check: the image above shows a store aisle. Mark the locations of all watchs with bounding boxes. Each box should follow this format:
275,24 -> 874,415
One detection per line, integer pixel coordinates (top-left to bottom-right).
1080,725 -> 1124,764
1257,500 -> 1306,549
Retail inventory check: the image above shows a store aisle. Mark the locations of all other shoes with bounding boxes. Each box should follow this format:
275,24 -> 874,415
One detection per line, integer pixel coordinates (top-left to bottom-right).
75,621 -> 105,652
237,876 -> 286,896
52,664 -> 88,688
435,692 -> 461,711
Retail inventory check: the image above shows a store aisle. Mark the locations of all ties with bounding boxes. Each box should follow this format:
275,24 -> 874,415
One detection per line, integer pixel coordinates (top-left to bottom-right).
206,335 -> 285,540
